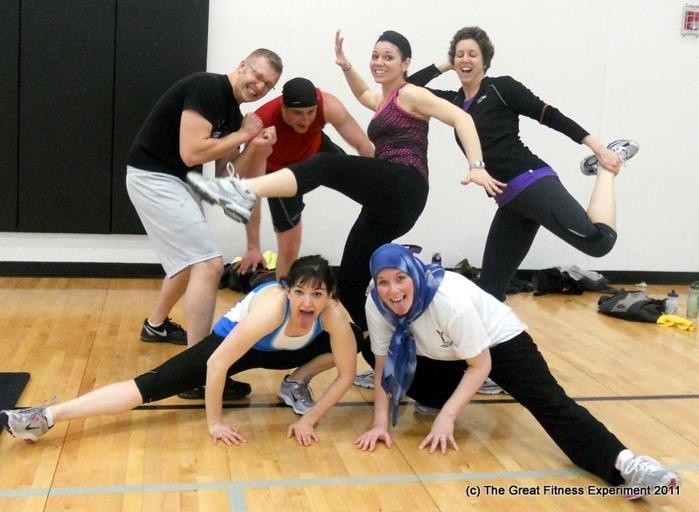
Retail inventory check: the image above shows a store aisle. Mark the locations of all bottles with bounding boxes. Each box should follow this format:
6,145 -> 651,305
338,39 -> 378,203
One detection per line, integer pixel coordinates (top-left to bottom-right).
687,281 -> 699,318
432,253 -> 442,266
665,290 -> 679,314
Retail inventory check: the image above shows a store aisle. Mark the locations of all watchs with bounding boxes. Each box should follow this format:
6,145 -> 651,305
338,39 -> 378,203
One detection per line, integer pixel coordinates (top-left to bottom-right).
469,160 -> 486,169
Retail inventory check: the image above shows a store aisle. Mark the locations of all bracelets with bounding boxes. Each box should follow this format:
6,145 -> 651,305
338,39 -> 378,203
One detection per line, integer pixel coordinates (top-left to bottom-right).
342,63 -> 352,71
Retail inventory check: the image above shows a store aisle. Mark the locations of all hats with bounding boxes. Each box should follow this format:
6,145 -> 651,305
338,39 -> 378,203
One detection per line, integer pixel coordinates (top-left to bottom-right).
282,77 -> 318,108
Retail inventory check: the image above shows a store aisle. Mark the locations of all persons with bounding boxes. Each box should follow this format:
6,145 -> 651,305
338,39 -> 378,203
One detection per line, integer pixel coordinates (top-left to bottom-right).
233,78 -> 374,281
354,243 -> 677,501
186,29 -> 507,391
0,254 -> 364,446
126,48 -> 284,402
406,26 -> 640,394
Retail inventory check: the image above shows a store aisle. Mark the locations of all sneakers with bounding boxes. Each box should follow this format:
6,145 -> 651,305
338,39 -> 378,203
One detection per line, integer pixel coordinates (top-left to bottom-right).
141,316 -> 188,345
353,369 -> 374,388
479,377 -> 508,395
277,372 -> 314,415
580,138 -> 639,175
186,162 -> 256,224
618,453 -> 679,502
181,379 -> 250,402
0,396 -> 60,443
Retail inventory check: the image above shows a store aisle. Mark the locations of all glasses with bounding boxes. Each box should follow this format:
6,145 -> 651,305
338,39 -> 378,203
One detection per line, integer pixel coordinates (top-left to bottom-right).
245,59 -> 275,92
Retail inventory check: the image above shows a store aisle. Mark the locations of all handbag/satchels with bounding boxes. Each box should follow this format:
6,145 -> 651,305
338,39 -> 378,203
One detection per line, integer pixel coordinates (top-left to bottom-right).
531,266 -> 564,293
599,288 -> 666,324
220,261 -> 276,295
